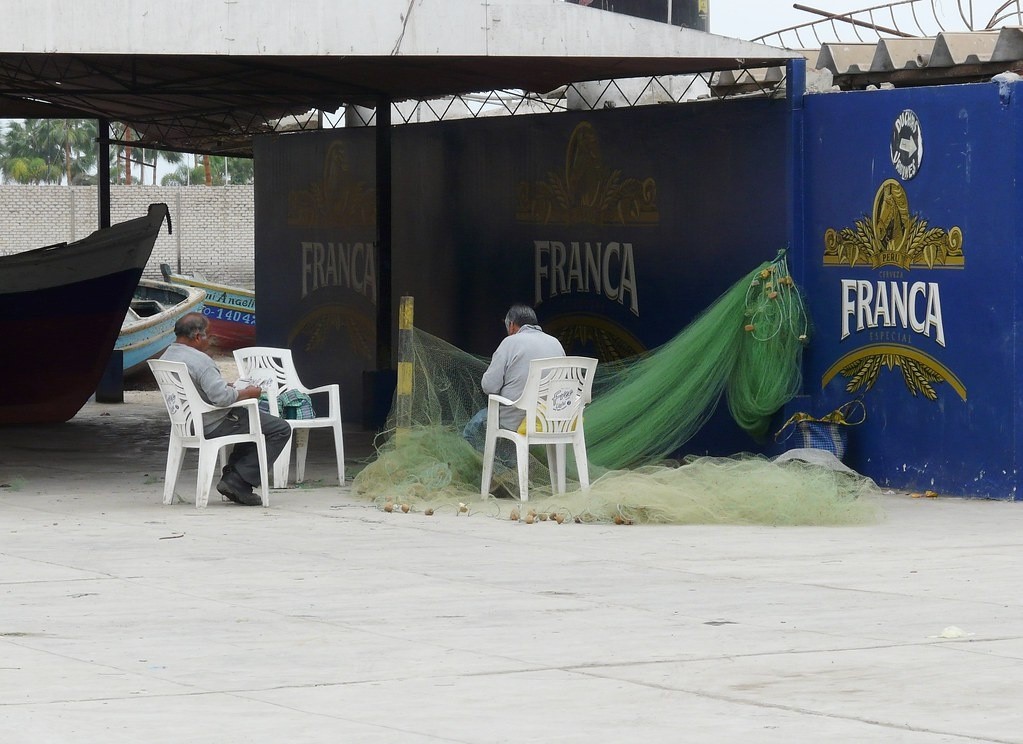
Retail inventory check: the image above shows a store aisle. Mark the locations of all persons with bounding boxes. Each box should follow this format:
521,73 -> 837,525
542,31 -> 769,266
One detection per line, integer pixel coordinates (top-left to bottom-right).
462,304 -> 567,493
158,313 -> 292,506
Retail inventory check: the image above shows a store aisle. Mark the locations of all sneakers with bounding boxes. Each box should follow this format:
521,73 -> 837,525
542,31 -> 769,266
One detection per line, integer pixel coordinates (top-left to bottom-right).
216,481 -> 263,506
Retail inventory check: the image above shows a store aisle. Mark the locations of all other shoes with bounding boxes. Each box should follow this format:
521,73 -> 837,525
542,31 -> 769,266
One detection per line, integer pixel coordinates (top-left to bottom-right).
489,470 -> 514,496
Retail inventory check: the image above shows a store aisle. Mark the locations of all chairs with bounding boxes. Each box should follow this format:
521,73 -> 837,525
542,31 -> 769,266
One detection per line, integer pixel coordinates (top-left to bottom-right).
477,356 -> 600,506
146,359 -> 270,511
232,346 -> 345,490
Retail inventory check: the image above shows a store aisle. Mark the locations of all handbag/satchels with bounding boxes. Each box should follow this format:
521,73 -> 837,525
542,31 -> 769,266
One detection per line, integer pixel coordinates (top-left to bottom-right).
259,389 -> 317,420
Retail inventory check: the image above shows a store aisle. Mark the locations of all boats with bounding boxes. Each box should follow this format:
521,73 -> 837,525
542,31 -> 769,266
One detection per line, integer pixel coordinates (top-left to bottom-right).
111,277 -> 206,381
1,201 -> 174,431
159,263 -> 256,351
